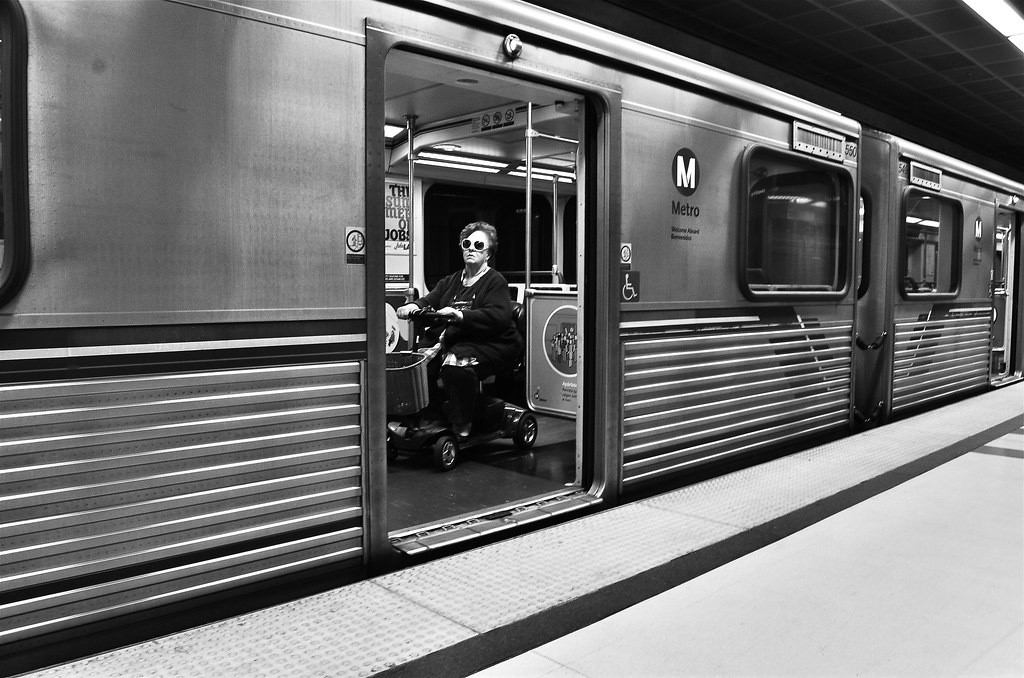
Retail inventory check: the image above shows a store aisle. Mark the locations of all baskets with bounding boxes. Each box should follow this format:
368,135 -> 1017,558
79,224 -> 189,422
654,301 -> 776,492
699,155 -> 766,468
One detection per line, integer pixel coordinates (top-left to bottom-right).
385,351 -> 429,415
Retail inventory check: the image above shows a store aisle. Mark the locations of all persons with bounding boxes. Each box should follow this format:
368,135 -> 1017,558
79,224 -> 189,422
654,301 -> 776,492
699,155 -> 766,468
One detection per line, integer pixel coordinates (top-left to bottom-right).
549,332 -> 577,363
396,221 -> 523,441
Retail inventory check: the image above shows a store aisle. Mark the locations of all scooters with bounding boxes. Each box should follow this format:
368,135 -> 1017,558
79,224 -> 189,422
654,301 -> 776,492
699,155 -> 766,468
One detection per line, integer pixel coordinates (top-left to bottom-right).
385,299 -> 538,472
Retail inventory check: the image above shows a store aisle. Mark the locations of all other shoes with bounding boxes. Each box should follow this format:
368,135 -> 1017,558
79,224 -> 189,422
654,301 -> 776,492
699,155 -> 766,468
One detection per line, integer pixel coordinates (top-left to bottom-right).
453,421 -> 472,436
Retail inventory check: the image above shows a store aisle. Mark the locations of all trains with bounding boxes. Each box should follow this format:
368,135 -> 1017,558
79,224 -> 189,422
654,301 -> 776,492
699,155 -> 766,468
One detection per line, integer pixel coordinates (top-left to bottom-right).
0,1 -> 1022,678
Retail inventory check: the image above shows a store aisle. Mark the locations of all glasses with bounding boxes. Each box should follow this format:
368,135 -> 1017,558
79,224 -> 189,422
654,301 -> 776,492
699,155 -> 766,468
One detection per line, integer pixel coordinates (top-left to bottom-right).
461,239 -> 490,252
